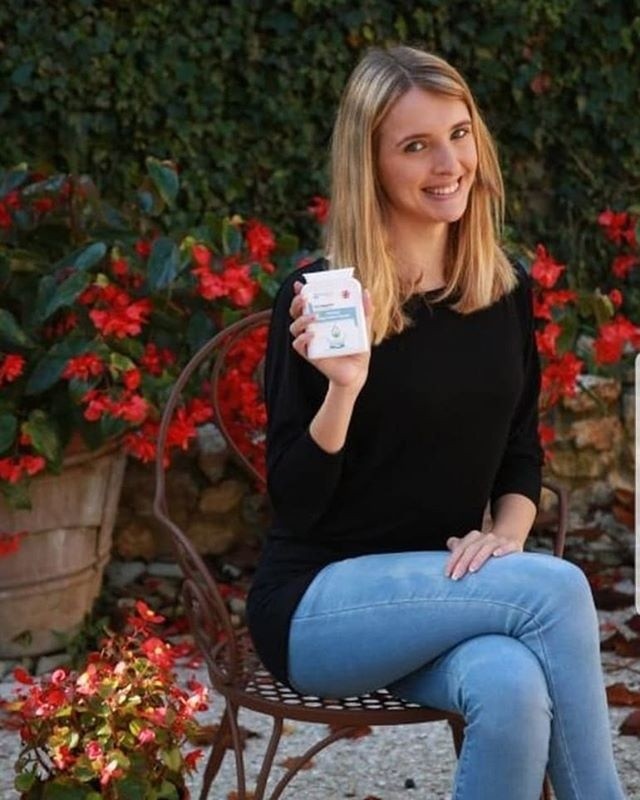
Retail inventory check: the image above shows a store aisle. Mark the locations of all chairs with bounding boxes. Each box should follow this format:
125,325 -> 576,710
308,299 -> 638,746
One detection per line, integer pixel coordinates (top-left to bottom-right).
153,307 -> 570,799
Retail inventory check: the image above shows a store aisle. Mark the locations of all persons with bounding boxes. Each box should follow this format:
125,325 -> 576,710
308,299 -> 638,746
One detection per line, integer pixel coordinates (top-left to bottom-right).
244,39 -> 632,800
